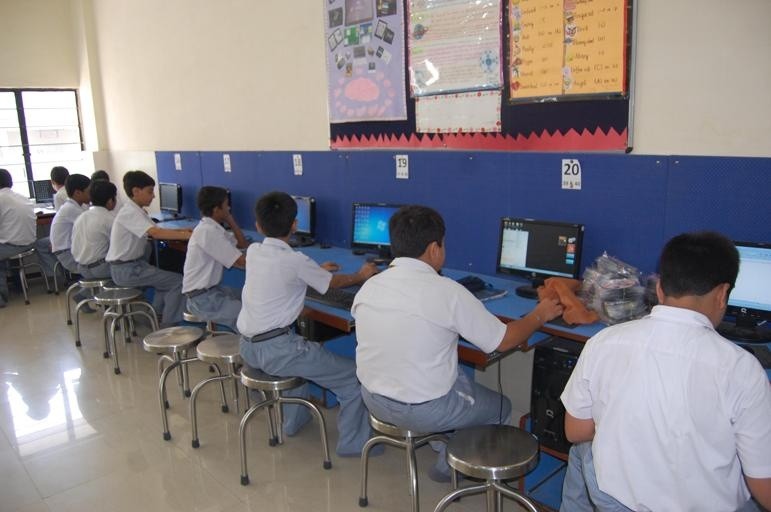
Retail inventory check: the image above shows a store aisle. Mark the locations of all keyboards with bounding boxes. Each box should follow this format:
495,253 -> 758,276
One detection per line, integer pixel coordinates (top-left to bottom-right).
306,285 -> 354,309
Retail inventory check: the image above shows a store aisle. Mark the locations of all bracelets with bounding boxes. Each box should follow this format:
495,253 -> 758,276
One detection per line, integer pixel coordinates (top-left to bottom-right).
528,312 -> 545,328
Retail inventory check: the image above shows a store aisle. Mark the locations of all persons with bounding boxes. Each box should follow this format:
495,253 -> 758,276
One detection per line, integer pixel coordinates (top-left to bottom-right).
349,203 -> 566,483
182,186 -> 250,337
235,189 -> 387,459
557,233 -> 771,512
0,166 -> 193,330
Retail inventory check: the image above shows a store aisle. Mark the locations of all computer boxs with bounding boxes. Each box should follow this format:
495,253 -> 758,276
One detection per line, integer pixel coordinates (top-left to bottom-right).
296,318 -> 341,341
531,337 -> 585,454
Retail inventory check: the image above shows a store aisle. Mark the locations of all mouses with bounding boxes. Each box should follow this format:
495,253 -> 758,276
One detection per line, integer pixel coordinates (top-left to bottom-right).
737,344 -> 755,355
152,218 -> 159,223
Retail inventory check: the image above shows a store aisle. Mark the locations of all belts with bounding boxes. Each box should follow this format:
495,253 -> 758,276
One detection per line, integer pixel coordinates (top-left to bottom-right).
88,257 -> 106,268
107,259 -> 132,266
185,288 -> 205,298
244,327 -> 287,343
53,247 -> 69,257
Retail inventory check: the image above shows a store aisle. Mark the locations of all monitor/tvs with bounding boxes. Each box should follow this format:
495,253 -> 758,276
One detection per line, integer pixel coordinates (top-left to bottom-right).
33,180 -> 57,209
288,195 -> 315,247
716,240 -> 771,343
497,217 -> 585,298
351,202 -> 403,264
159,183 -> 186,221
